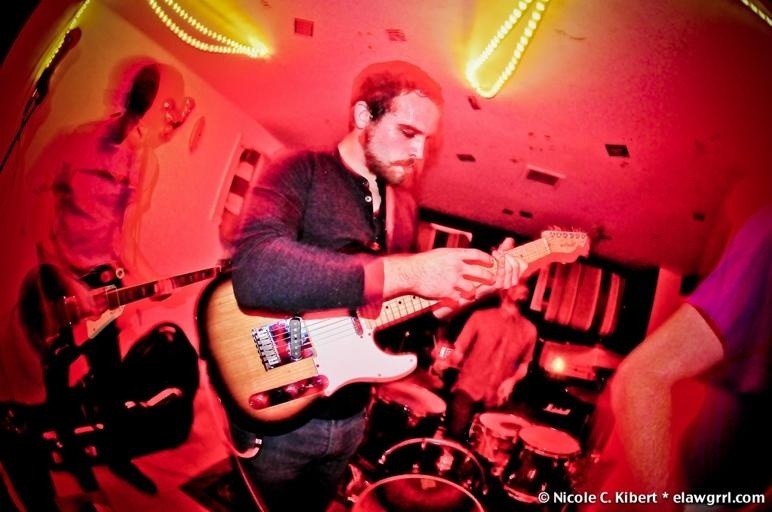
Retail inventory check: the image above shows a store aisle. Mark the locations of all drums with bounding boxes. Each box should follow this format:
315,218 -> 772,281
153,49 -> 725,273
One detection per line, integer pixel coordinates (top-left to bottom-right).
359,380 -> 448,467
499,426 -> 581,503
469,412 -> 529,467
352,438 -> 490,511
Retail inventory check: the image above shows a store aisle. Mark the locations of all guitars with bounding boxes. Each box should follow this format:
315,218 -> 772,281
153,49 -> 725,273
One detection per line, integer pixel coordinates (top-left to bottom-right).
20,262 -> 231,356
197,228 -> 592,432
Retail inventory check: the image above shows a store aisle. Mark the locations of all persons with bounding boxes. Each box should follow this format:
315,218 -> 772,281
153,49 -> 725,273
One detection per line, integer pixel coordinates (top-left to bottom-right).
433,276 -> 540,438
215,56 -> 530,511
576,215 -> 771,512
2,52 -> 175,501
